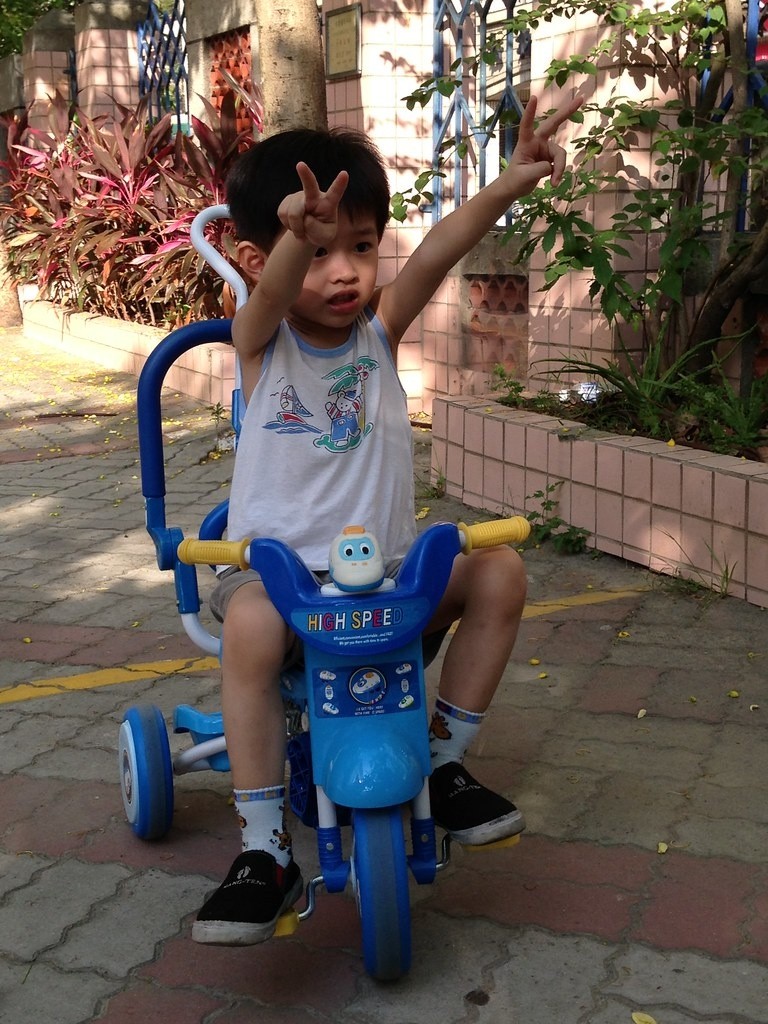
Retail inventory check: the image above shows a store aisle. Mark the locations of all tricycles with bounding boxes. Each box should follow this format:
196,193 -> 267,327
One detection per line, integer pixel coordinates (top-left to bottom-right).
116,200 -> 532,985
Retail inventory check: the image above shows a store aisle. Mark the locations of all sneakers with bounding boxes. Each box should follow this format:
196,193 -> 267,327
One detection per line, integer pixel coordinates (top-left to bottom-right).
428,761 -> 528,845
192,849 -> 304,946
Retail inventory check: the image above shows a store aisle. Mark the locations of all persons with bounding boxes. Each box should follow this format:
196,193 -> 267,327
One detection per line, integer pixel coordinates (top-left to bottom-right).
191,90 -> 586,945
